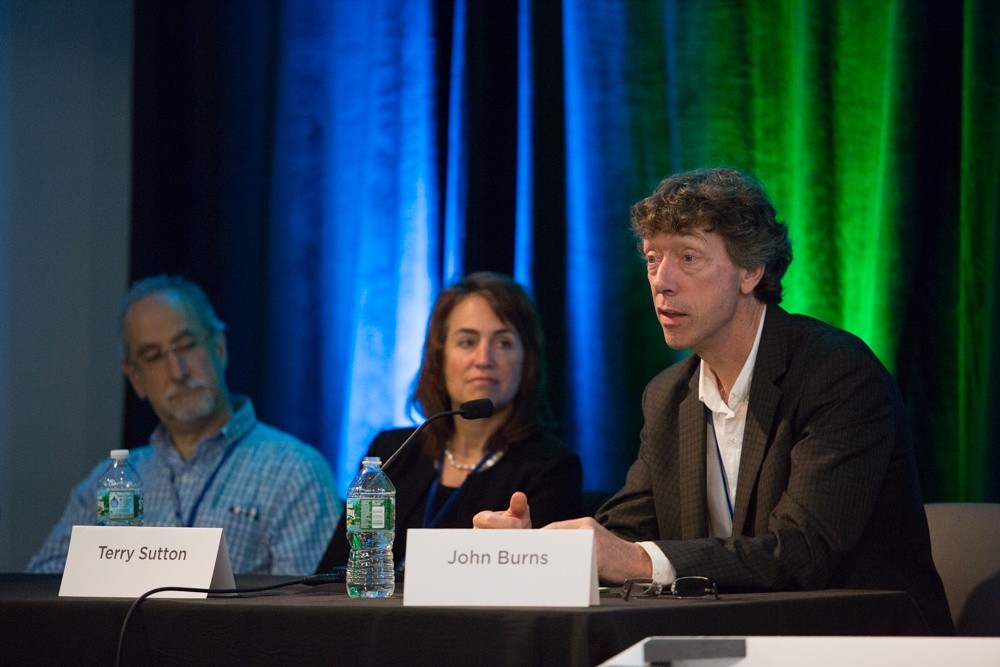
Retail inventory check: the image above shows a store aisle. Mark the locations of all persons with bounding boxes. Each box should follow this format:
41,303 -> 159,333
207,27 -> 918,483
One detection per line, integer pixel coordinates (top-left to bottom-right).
471,168 -> 961,638
327,271 -> 586,571
20,273 -> 344,577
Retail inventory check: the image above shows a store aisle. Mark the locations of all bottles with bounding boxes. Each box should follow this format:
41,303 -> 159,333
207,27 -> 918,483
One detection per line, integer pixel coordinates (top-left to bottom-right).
96,449 -> 145,527
346,457 -> 396,598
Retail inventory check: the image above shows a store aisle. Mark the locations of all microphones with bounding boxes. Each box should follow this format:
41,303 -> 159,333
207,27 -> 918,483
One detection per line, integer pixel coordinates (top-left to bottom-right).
365,397 -> 494,491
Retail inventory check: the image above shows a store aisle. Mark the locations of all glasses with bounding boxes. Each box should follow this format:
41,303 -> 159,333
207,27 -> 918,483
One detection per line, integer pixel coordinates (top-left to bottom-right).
131,324 -> 216,370
618,576 -> 724,600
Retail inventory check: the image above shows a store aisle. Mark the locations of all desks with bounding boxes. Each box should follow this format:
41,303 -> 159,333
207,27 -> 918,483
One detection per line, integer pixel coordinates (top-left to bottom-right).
0,571 -> 919,667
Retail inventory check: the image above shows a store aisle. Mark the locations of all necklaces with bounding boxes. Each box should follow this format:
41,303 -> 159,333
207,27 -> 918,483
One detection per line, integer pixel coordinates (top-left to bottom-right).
447,440 -> 477,469
